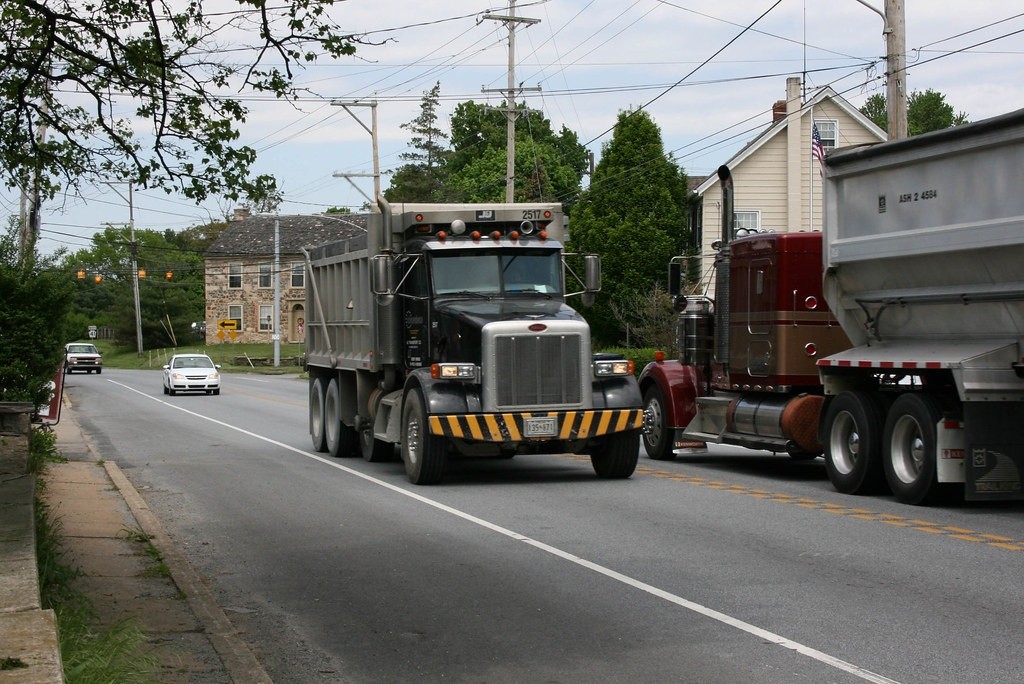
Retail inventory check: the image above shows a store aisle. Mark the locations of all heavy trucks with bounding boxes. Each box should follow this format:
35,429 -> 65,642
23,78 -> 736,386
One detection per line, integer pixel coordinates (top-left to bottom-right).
295,199 -> 646,486
639,105 -> 1023,501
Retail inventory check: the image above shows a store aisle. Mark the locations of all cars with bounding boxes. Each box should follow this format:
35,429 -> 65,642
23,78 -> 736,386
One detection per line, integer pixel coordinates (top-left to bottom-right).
63,343 -> 102,374
162,353 -> 221,396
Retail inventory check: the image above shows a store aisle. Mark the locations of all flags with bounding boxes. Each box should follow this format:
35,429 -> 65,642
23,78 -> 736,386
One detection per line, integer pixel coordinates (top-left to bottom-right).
812,121 -> 825,180
